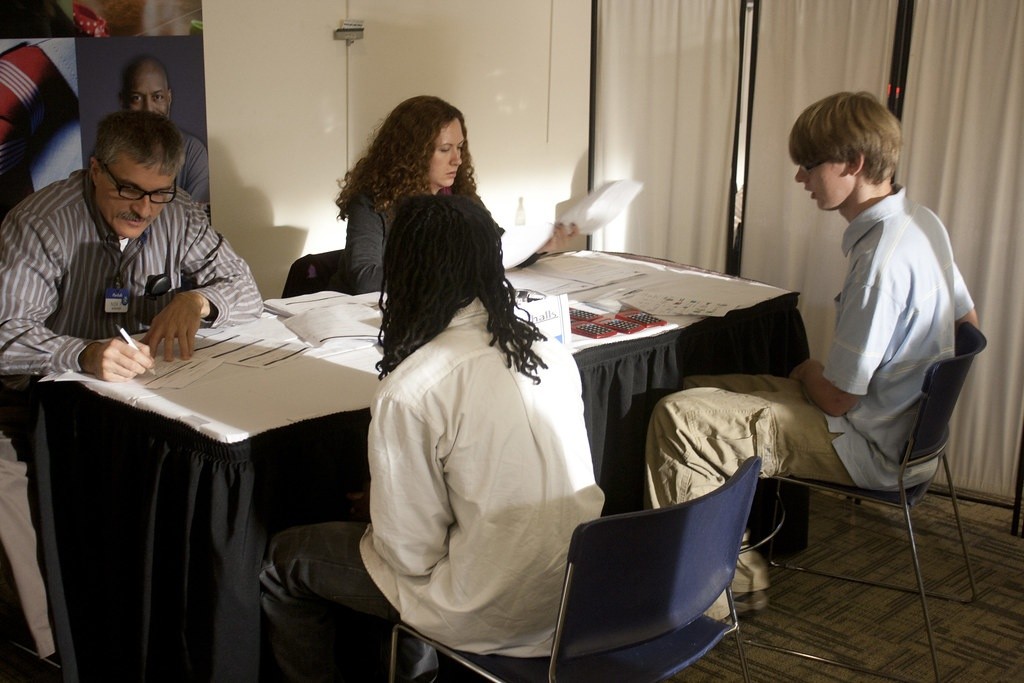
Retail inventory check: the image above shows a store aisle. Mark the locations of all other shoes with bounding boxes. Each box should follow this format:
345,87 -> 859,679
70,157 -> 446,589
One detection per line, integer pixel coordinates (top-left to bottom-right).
731,591 -> 769,613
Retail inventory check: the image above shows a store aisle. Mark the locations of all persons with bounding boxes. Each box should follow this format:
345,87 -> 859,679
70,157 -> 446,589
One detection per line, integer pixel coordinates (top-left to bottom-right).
644,92 -> 979,625
260,196 -> 605,683
335,95 -> 580,296
0,110 -> 264,658
118,54 -> 210,204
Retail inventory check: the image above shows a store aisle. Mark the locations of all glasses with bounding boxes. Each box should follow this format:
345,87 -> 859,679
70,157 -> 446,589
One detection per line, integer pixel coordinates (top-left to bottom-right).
801,159 -> 825,175
103,164 -> 177,204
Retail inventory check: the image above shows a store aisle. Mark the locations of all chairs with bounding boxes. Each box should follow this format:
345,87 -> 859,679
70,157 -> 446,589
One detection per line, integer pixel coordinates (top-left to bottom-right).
388,455 -> 764,683
728,321 -> 987,683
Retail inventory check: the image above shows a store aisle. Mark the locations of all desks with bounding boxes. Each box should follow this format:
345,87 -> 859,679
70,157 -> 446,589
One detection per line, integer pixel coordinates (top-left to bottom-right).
32,252 -> 812,683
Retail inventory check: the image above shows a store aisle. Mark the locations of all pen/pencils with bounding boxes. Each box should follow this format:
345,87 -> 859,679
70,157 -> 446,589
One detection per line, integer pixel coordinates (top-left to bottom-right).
116,323 -> 158,378
